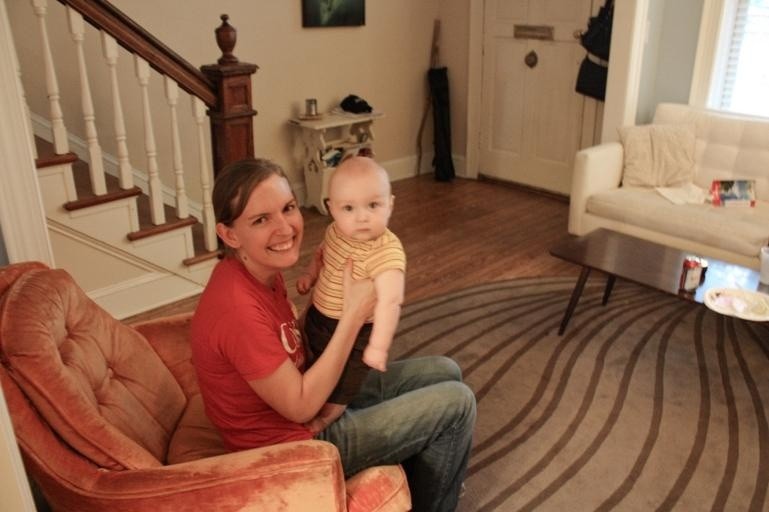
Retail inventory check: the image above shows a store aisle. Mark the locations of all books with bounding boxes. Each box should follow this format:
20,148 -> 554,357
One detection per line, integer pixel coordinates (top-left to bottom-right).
704,179 -> 757,209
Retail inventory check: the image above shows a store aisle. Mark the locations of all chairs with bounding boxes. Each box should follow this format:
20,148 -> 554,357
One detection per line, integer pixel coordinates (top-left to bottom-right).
0,260 -> 414,511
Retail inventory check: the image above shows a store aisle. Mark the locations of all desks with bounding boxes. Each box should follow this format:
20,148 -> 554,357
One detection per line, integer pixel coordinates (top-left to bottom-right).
289,105 -> 384,216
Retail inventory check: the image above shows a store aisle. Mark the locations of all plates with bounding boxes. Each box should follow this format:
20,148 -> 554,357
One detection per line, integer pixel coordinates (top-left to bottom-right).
704,288 -> 769,322
299,113 -> 325,121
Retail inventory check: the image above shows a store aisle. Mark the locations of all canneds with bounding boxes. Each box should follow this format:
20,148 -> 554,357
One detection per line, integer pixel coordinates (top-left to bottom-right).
680,256 -> 702,291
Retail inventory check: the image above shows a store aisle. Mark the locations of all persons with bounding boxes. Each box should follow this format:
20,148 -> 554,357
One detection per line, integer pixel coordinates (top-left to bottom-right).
190,156 -> 478,512
296,155 -> 408,434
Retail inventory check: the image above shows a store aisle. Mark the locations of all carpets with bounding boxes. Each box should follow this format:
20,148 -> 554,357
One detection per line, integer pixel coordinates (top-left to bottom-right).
354,270 -> 769,511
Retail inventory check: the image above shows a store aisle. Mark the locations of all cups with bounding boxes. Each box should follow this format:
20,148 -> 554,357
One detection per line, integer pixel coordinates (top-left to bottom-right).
759,247 -> 769,285
305,99 -> 318,116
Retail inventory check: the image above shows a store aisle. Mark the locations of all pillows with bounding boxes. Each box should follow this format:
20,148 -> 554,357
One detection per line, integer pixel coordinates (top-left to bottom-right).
615,119 -> 697,190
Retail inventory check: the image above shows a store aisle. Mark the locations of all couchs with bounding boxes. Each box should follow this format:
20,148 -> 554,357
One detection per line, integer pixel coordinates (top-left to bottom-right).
566,101 -> 768,267
